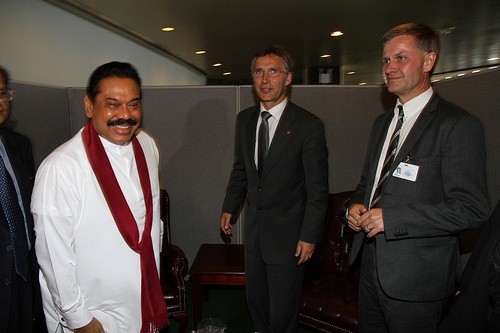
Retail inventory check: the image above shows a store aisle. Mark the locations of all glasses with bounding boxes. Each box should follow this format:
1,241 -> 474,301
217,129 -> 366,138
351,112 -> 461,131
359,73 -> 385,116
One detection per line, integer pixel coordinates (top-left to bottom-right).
252,67 -> 288,76
0,88 -> 17,102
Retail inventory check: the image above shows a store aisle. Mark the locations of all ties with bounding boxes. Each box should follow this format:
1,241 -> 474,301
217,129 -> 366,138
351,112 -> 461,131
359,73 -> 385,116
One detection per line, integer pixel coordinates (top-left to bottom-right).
370,104 -> 404,210
258,110 -> 271,178
0,154 -> 30,282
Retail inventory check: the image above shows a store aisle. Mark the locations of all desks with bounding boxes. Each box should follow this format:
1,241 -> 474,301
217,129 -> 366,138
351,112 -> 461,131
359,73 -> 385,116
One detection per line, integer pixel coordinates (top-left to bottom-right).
190,243 -> 246,333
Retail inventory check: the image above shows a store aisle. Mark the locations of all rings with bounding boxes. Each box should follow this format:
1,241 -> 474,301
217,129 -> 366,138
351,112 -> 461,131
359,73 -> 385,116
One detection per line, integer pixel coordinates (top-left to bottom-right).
366,224 -> 372,231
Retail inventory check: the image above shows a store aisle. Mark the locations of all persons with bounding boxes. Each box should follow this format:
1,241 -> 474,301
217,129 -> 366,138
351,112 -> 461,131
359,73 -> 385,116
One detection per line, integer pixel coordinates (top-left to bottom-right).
30,62 -> 169,333
219,45 -> 329,333
342,22 -> 491,333
0,66 -> 49,333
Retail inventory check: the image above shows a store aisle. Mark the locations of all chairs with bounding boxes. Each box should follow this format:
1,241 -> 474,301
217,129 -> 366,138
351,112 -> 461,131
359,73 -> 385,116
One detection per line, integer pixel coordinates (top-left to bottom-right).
298,191 -> 359,333
159,188 -> 190,333
